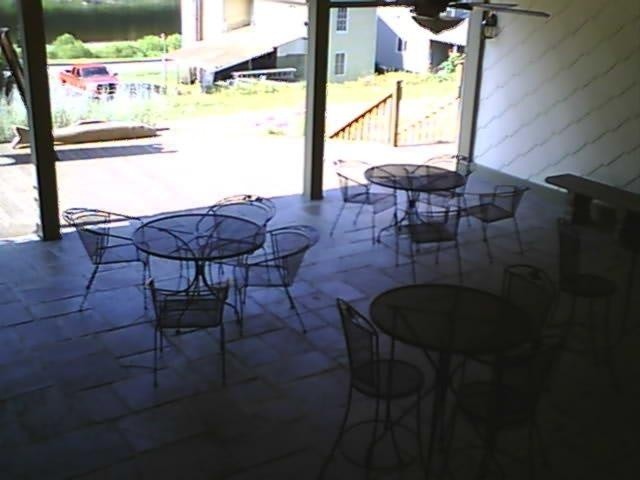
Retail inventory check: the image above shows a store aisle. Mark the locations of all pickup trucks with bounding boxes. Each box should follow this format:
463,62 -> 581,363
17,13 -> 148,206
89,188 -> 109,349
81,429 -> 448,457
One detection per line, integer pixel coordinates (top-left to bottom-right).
57,64 -> 122,102
